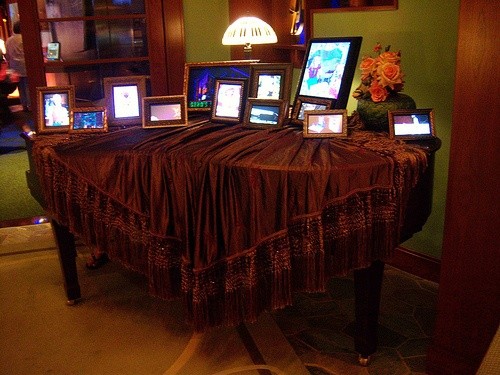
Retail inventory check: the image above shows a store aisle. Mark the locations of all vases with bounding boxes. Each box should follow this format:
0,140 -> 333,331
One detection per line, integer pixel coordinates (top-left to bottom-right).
357,94 -> 416,130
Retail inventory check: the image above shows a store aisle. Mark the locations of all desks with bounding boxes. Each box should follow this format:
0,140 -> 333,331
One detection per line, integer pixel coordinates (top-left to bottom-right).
25,115 -> 441,366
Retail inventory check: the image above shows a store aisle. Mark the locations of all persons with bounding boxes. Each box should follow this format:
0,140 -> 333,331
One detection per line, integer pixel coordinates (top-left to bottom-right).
6,21 -> 32,113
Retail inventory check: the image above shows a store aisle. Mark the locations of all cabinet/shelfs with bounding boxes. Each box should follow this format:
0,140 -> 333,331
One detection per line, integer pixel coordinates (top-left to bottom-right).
18,0 -> 187,116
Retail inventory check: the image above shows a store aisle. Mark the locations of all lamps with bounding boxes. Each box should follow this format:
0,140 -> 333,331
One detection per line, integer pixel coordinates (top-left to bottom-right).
221,15 -> 277,60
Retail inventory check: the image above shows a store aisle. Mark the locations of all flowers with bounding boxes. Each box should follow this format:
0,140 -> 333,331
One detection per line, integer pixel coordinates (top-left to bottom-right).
352,41 -> 407,103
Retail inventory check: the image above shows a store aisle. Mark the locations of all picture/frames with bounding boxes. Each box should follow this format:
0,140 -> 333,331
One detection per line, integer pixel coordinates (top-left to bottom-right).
141,95 -> 189,129
210,78 -> 246,125
103,76 -> 146,126
303,110 -> 348,138
69,106 -> 108,134
292,36 -> 363,110
388,108 -> 436,141
246,62 -> 292,126
241,98 -> 287,131
183,60 -> 259,112
35,85 -> 75,135
291,96 -> 332,126
